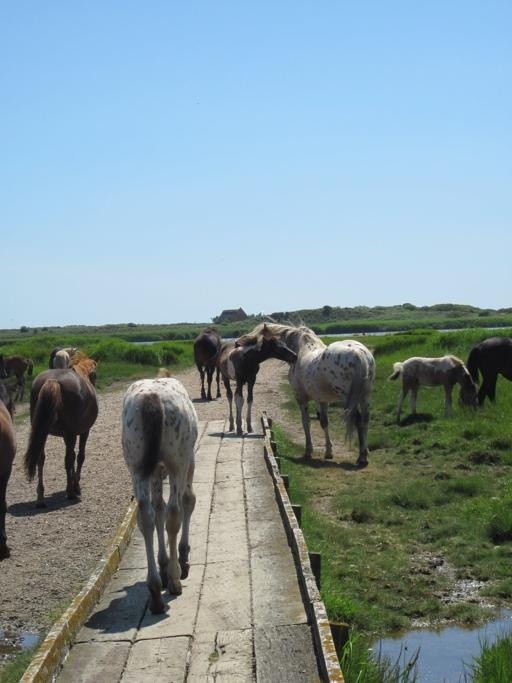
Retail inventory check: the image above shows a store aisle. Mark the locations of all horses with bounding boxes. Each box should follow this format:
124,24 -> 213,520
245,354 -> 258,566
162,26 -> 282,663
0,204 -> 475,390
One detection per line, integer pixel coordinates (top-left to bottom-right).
120,367 -> 197,615
388,354 -> 479,425
466,335 -> 512,409
0,344 -> 101,563
193,316 -> 377,469
